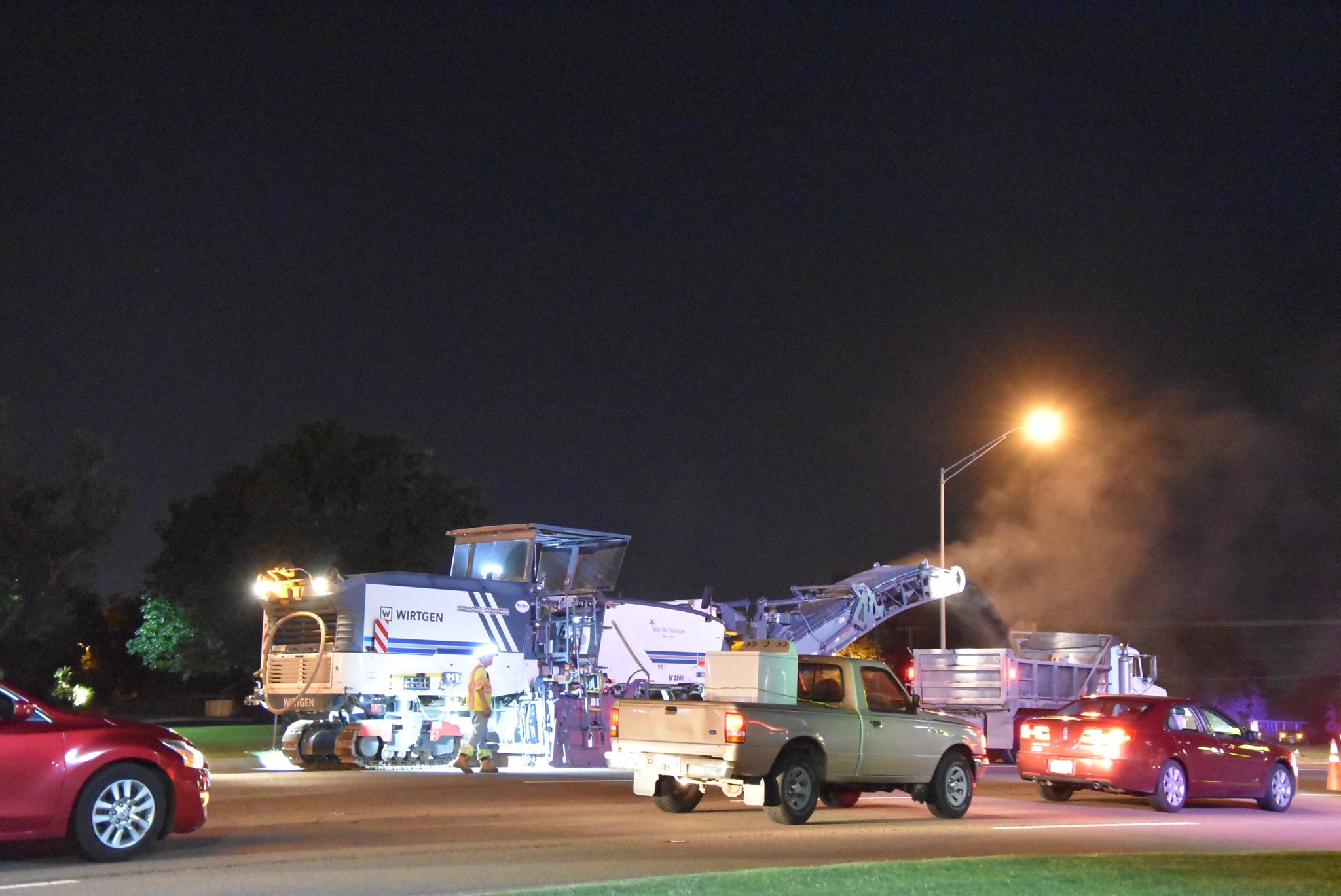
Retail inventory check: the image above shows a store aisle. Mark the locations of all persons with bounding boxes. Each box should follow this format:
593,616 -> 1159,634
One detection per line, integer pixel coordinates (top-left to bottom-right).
453,654 -> 500,774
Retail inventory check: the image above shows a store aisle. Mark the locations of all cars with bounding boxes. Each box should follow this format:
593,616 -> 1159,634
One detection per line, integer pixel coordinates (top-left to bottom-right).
0,679 -> 211,864
1016,693 -> 1298,813
1278,729 -> 1303,747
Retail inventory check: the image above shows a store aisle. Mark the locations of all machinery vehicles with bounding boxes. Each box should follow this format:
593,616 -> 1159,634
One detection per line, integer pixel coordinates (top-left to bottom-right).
244,521 -> 732,772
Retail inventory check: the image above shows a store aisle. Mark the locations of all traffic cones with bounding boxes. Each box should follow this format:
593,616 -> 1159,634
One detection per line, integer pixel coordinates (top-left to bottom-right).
1324,738 -> 1341,794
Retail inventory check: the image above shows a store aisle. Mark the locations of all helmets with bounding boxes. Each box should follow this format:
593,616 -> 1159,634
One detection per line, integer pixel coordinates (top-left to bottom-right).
472,644 -> 499,660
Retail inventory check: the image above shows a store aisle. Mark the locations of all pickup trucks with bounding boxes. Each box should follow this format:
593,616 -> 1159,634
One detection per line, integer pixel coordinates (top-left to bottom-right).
604,637 -> 991,827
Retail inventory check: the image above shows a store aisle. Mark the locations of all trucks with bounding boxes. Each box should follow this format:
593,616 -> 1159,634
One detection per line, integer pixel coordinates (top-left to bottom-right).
909,629 -> 1169,766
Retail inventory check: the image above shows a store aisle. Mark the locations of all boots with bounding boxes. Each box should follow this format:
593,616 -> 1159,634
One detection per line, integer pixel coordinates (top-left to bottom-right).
477,750 -> 498,772
453,744 -> 475,773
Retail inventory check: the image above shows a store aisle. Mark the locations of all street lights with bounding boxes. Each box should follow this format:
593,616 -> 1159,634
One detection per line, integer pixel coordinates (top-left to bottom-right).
939,406 -> 1064,650
